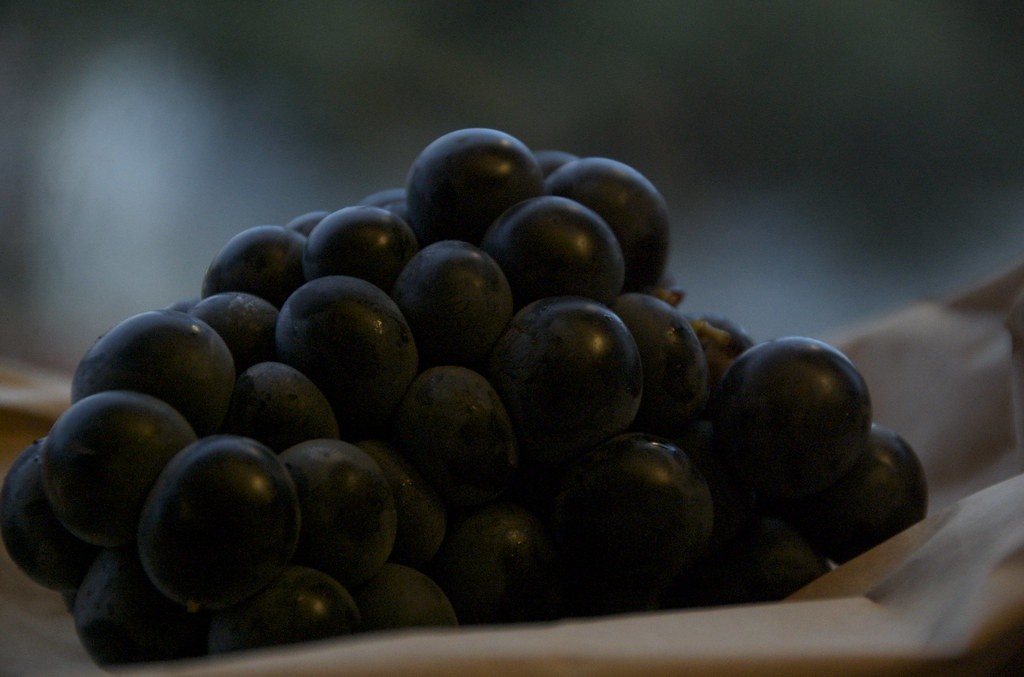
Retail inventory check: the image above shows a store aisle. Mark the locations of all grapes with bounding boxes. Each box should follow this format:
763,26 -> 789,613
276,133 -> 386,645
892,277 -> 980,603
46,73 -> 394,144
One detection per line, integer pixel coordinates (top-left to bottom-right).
1,128 -> 931,667
